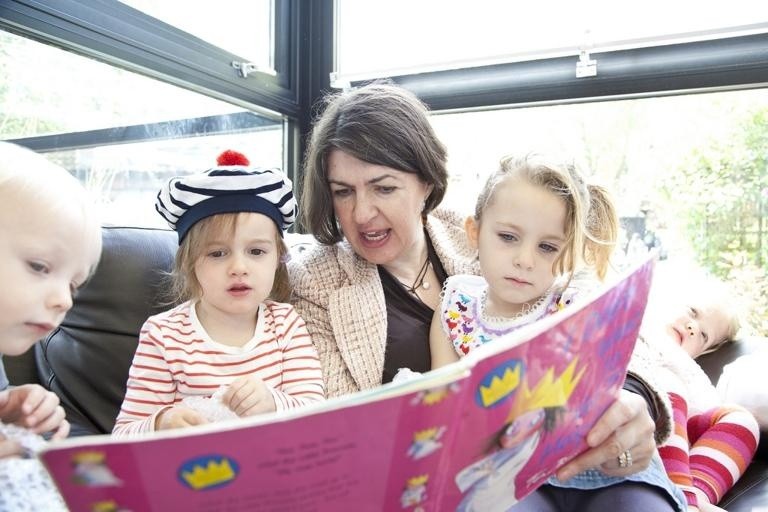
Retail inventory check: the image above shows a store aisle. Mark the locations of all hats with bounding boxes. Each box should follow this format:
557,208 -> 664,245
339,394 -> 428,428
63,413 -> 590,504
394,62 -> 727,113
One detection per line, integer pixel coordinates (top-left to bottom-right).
154,150 -> 300,245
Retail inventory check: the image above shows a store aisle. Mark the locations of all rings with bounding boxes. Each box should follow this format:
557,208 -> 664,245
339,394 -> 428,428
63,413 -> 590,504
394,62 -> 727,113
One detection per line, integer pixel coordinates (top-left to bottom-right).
616,450 -> 632,469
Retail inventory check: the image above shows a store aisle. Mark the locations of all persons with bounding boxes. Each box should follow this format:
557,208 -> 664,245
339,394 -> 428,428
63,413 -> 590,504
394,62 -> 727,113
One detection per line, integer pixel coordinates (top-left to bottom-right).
427,147 -> 688,512
0,139 -> 103,512
641,278 -> 761,512
281,83 -> 676,512
110,147 -> 326,438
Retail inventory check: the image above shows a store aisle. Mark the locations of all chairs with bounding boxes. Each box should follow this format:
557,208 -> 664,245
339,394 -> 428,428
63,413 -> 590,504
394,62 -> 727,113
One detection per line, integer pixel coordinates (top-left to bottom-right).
36,220 -> 768,512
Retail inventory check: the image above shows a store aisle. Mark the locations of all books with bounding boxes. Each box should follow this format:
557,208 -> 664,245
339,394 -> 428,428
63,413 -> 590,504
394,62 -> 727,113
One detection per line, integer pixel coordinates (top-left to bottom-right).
40,252 -> 658,512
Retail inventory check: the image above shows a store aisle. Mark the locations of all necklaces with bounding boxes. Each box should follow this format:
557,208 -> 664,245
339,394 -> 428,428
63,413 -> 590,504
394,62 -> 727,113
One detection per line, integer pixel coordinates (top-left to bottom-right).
396,256 -> 435,307
